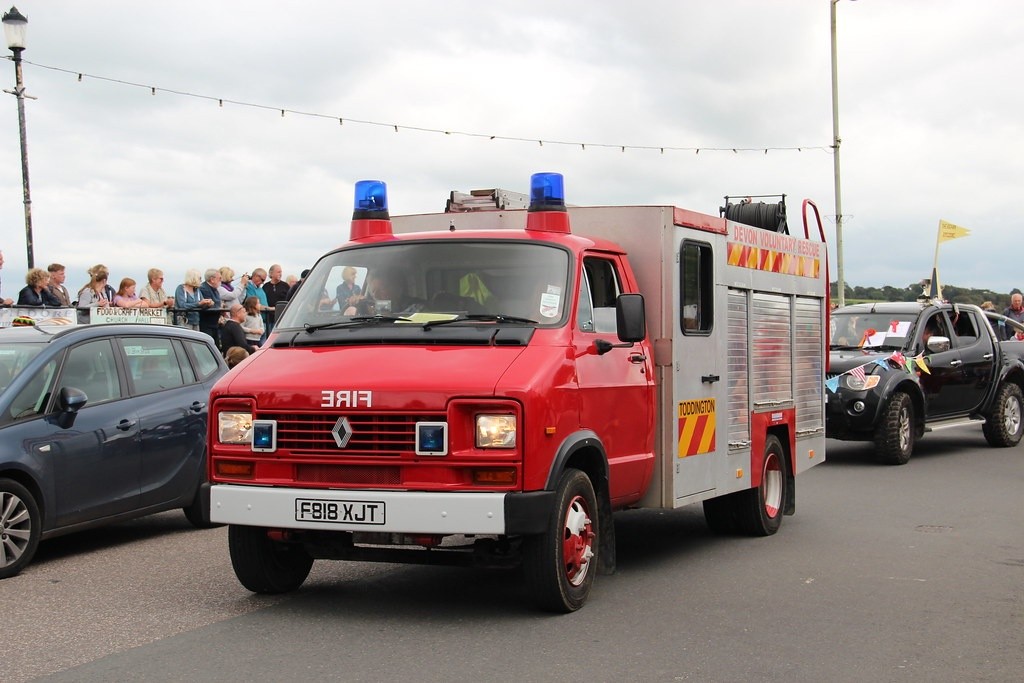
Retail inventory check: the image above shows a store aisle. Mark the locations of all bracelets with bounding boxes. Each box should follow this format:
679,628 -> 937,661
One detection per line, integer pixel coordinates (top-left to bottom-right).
164,301 -> 167,305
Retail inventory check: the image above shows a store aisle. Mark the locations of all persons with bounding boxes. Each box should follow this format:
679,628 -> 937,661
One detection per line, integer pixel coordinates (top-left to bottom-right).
17,264 -> 71,307
173,265 -> 331,370
77,264 -> 174,309
335,267 -> 361,310
346,265 -> 426,313
982,294 -> 1024,340
0,250 -> 14,305
923,323 -> 935,342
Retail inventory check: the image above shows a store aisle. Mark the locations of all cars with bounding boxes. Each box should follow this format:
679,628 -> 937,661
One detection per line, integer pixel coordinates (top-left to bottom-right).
1,322 -> 232,579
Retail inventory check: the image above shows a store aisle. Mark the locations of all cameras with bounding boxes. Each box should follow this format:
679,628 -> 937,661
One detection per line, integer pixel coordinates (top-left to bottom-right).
243,275 -> 252,280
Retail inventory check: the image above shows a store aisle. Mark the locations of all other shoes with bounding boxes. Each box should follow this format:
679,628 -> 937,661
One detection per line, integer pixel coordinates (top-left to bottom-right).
925,426 -> 932,432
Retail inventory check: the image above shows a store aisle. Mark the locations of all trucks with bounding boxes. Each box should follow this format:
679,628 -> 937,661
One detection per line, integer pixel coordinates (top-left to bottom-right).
201,171 -> 833,614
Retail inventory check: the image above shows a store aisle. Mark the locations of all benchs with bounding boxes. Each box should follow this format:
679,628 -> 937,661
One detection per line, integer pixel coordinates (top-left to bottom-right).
87,371 -> 183,401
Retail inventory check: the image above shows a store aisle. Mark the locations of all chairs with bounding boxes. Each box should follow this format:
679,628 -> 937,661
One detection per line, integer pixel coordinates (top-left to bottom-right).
431,291 -> 458,310
65,358 -> 109,405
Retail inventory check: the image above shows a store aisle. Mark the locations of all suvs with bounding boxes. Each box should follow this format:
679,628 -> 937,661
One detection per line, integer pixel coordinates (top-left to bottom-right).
825,299 -> 1024,465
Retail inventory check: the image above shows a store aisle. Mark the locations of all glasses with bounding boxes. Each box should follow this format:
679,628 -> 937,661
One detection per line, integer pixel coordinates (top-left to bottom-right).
154,278 -> 163,282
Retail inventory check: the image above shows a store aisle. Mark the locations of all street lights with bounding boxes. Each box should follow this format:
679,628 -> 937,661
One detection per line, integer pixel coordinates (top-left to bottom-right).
1,5 -> 36,271
